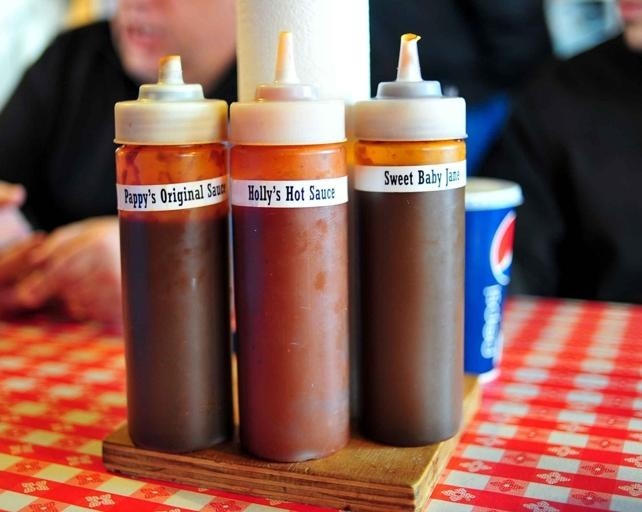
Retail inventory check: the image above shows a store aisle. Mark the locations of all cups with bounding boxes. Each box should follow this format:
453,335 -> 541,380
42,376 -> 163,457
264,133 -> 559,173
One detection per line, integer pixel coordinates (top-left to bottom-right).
464,174 -> 524,375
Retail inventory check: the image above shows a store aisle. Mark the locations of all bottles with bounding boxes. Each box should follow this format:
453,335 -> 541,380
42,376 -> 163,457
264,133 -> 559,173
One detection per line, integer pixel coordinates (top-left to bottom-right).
352,30 -> 466,447
227,30 -> 354,462
113,55 -> 235,455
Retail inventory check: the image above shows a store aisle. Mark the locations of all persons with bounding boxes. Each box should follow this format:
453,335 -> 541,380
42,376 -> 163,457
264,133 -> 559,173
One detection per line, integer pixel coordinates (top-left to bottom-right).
0,1 -> 237,337
474,0 -> 641,307
